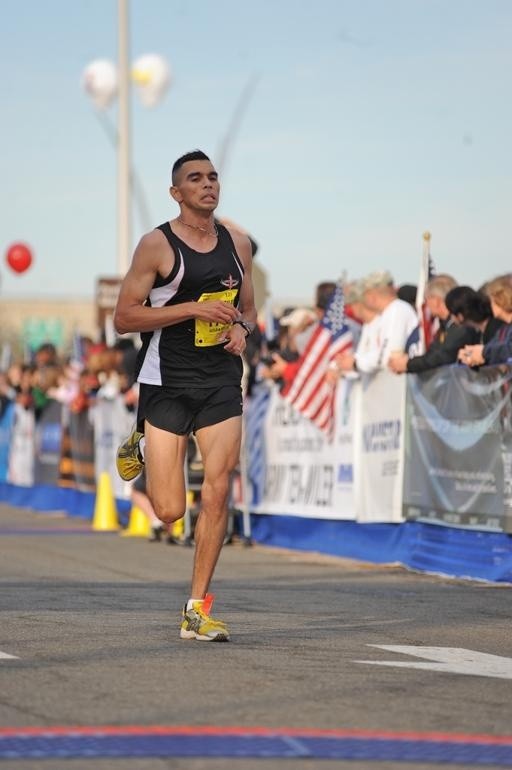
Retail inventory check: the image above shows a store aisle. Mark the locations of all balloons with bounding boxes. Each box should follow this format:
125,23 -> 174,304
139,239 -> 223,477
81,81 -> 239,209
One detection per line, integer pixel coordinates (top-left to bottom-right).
5,240 -> 34,275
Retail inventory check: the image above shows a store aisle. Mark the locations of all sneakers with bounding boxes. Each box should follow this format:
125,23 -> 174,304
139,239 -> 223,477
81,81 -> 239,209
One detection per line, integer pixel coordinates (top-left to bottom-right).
177,595 -> 234,643
115,418 -> 147,482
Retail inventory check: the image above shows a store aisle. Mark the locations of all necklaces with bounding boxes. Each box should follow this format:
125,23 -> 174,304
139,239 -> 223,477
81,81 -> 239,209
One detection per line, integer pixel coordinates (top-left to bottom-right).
175,218 -> 218,238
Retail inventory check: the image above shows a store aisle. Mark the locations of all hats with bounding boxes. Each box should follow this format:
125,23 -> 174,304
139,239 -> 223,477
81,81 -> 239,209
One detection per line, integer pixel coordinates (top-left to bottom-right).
277,307 -> 319,327
341,269 -> 394,307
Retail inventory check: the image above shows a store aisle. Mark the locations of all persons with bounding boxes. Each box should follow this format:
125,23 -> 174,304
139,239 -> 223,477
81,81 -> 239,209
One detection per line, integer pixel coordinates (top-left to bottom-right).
112,149 -> 257,642
2,269 -> 512,553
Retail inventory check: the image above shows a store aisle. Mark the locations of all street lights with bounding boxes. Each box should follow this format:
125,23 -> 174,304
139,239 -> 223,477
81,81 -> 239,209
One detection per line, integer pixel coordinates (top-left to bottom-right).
82,50 -> 170,276
